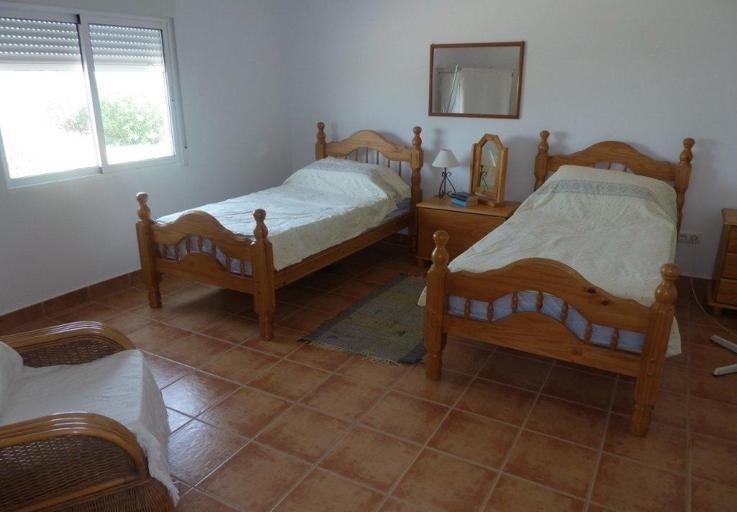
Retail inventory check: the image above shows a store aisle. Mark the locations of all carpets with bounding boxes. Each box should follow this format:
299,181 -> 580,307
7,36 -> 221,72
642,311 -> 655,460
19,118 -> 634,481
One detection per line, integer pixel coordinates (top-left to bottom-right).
293,268 -> 431,369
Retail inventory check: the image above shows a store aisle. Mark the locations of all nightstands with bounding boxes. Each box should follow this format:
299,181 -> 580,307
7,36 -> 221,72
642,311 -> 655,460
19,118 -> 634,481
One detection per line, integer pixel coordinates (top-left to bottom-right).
703,207 -> 737,317
413,190 -> 521,271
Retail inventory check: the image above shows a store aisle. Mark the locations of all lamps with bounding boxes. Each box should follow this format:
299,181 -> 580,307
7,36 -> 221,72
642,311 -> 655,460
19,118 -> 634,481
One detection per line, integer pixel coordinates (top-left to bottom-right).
431,145 -> 461,202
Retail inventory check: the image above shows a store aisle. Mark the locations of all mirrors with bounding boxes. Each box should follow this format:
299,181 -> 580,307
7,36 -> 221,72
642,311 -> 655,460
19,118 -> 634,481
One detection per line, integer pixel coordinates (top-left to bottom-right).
467,132 -> 509,208
425,36 -> 527,124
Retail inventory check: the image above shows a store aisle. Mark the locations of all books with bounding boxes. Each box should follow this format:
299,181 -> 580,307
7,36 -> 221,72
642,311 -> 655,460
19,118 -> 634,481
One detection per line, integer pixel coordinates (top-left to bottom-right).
455,195 -> 477,202
451,198 -> 479,207
457,192 -> 480,197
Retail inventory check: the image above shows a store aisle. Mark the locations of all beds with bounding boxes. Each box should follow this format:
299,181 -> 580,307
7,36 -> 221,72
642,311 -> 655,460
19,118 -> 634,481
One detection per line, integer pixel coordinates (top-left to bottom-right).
415,123 -> 697,438
130,114 -> 425,350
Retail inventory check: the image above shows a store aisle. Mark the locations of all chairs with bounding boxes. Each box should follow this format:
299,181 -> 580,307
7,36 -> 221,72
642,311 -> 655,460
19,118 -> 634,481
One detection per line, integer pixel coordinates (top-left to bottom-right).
0,320 -> 175,511
